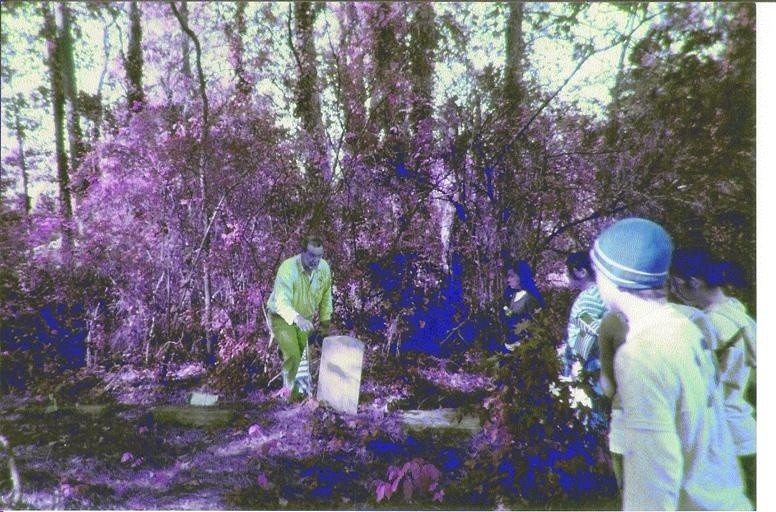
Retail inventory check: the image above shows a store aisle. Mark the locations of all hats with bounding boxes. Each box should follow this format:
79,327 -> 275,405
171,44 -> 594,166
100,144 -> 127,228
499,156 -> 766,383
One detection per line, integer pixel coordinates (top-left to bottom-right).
589,218 -> 673,289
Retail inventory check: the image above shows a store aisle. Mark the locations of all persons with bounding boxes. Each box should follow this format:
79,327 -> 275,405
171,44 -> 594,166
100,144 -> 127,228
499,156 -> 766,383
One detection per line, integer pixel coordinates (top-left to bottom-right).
586,216 -> 756,511
559,251 -> 613,433
665,244 -> 756,511
596,301 -> 723,504
264,237 -> 334,409
497,258 -> 546,354
